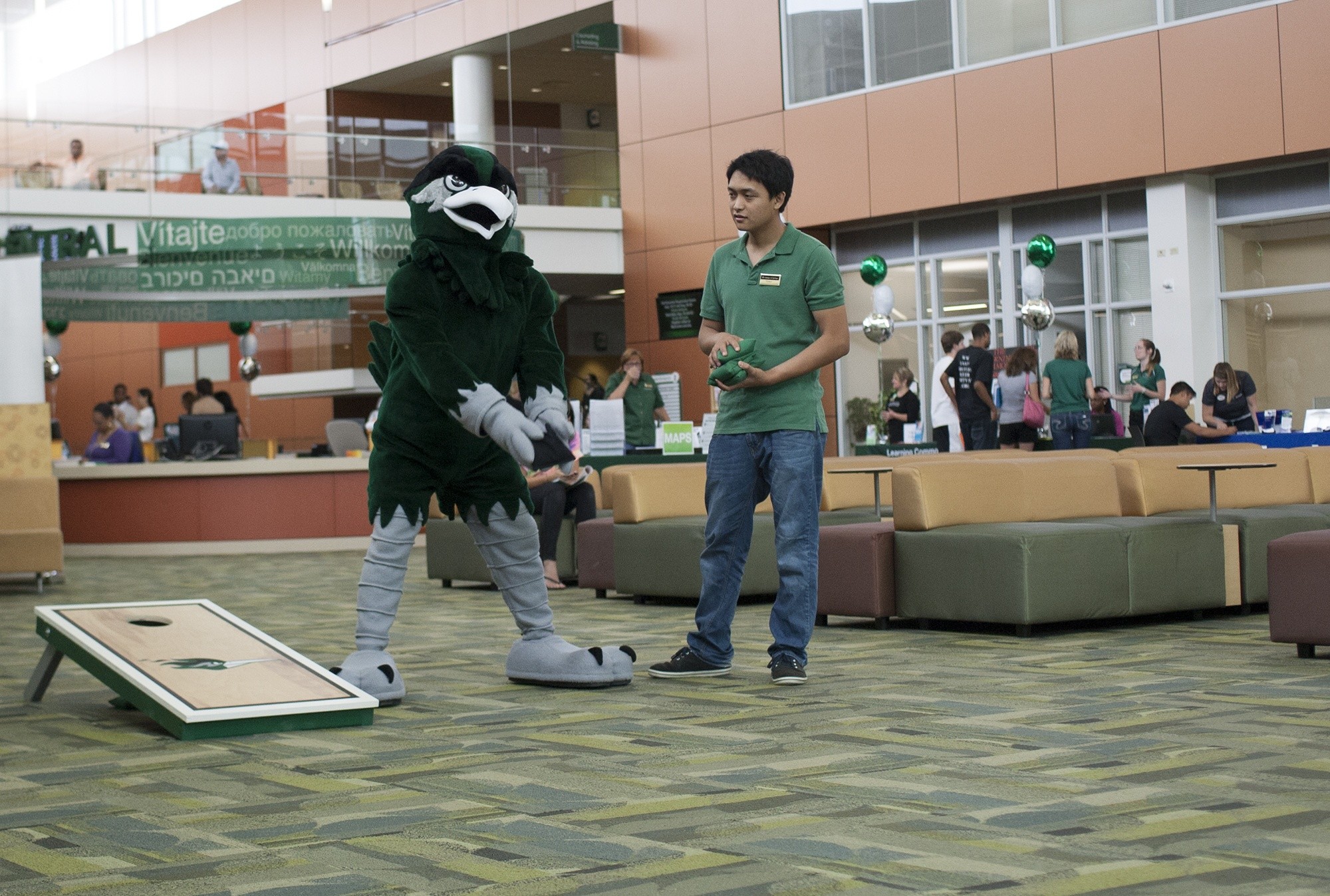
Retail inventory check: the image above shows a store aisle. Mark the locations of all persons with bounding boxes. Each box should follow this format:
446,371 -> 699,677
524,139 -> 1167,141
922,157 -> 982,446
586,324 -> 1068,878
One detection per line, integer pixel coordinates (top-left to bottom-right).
602,348 -> 670,446
997,347 -> 1051,450
1042,329 -> 1095,449
882,367 -> 921,443
1090,387 -> 1123,437
202,142 -> 241,192
940,323 -> 999,451
524,402 -> 596,591
586,412 -> 590,426
83,323 -> 1259,462
193,378 -> 225,415
648,151 -> 850,683
45,139 -> 102,188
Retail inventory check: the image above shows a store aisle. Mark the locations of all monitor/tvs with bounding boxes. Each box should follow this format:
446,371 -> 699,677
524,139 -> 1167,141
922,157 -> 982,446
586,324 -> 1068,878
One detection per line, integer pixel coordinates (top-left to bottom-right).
178,413 -> 239,461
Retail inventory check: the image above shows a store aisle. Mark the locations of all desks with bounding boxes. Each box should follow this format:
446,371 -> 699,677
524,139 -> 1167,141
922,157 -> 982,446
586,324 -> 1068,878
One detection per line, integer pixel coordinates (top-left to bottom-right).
827,467 -> 893,521
1178,463 -> 1277,603
54,457 -> 425,557
1198,432 -> 1330,449
856,443 -> 939,457
579,454 -> 707,476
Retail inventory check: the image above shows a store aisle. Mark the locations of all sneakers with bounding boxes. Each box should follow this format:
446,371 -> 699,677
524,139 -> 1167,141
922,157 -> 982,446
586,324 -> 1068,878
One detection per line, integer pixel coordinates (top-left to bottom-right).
647,647 -> 732,678
767,653 -> 807,685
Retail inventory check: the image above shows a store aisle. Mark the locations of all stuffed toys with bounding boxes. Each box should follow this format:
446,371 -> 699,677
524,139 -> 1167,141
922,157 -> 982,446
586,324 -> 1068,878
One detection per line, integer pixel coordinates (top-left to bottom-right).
325,146 -> 637,709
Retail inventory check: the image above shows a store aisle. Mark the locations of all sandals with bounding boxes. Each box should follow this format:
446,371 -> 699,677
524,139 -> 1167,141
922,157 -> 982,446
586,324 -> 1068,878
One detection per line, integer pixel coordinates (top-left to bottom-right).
544,576 -> 566,589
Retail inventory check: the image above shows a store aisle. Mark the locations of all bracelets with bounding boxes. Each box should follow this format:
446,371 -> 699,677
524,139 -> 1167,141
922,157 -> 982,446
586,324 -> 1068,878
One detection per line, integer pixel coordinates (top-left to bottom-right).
1140,387 -> 1146,393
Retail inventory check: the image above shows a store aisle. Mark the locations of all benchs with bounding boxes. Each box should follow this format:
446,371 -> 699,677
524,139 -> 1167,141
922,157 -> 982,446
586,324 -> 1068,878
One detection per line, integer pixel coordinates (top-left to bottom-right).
426,444 -> 1330,659
0,476 -> 63,591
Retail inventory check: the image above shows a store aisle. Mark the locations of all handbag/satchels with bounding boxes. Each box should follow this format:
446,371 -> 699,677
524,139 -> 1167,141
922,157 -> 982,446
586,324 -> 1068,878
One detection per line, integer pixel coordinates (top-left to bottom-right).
1023,372 -> 1044,428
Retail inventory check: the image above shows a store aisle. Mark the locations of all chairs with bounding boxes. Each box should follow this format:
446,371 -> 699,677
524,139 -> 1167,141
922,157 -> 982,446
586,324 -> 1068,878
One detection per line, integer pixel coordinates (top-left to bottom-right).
375,182 -> 402,199
340,181 -> 363,198
245,175 -> 261,194
326,420 -> 369,456
1128,425 -> 1145,446
